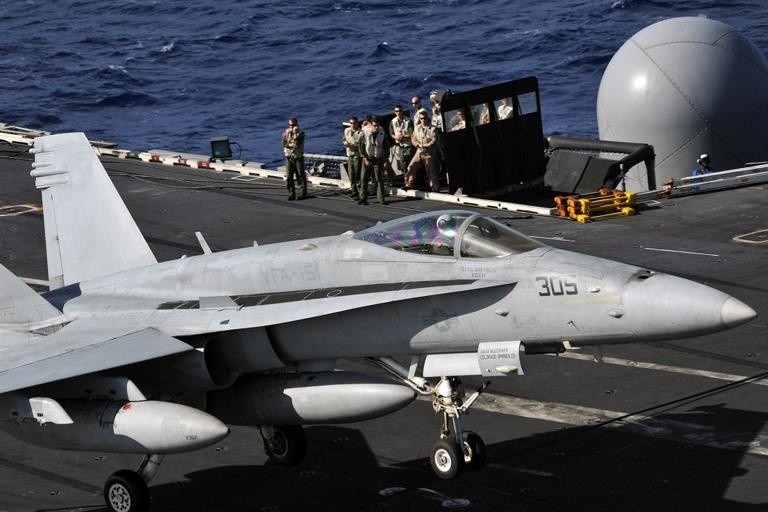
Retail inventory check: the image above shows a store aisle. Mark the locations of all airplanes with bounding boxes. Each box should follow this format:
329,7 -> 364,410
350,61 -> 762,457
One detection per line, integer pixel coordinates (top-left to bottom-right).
0,131 -> 758,510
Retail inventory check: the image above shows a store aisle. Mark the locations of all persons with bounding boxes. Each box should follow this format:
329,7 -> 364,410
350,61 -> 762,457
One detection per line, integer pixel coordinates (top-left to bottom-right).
688,153 -> 714,175
281,118 -> 307,200
431,214 -> 466,256
341,90 -> 514,207
